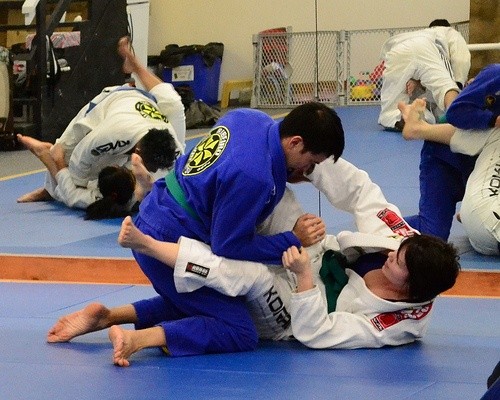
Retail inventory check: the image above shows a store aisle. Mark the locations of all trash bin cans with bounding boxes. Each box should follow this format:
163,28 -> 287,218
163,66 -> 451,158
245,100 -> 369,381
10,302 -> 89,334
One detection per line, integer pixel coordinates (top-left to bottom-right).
159,42 -> 225,114
147,54 -> 160,76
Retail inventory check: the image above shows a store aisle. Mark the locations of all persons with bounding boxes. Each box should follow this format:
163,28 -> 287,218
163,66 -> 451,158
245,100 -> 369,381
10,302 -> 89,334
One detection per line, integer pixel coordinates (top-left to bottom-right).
403,61 -> 500,244
16,37 -> 185,221
46,101 -> 345,367
397,97 -> 500,255
117,155 -> 462,350
377,18 -> 471,134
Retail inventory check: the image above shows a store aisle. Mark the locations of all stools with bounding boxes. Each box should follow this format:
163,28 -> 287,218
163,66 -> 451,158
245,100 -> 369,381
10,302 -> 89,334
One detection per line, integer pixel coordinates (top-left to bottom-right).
221,80 -> 253,109
